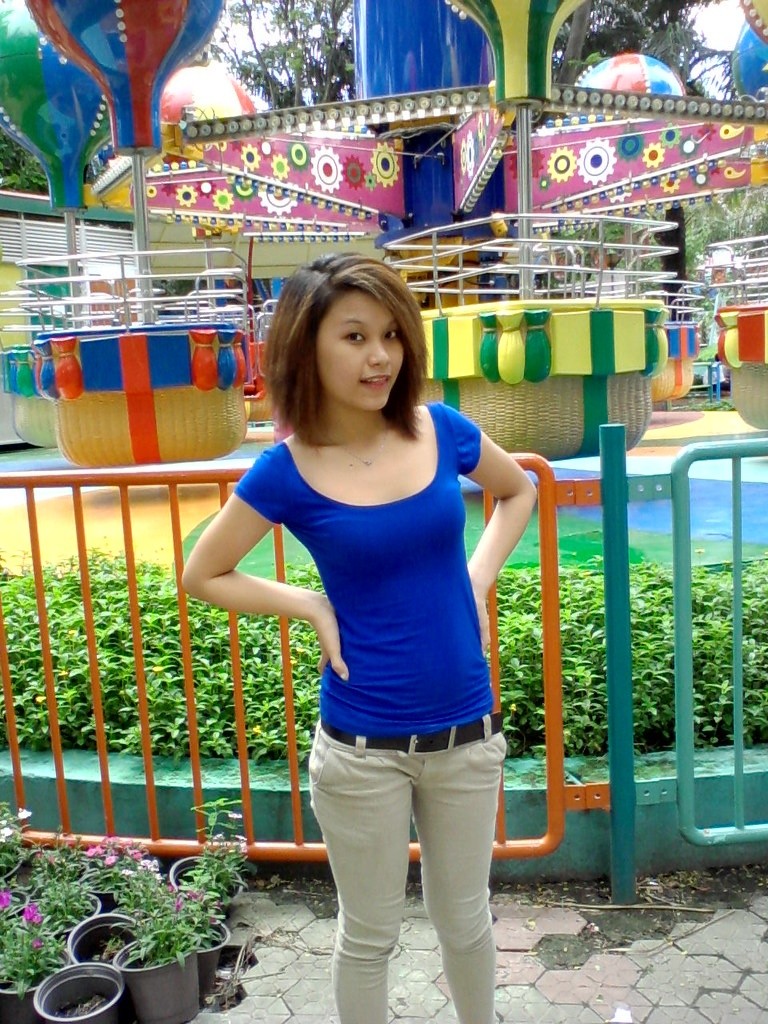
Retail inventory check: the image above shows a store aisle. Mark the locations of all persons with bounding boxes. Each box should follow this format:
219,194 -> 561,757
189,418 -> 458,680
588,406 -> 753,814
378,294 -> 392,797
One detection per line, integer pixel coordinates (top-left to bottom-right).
703,354 -> 731,396
180,251 -> 538,1024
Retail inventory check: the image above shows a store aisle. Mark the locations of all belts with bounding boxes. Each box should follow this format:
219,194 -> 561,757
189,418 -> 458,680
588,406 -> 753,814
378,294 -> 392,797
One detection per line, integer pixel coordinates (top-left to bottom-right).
320,712 -> 503,753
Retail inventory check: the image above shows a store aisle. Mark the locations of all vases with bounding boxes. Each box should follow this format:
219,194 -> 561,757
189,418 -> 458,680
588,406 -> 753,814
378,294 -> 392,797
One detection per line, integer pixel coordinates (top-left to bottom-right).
33,962 -> 126,1024
167,857 -> 243,911
0,946 -> 71,1024
195,922 -> 231,1001
113,940 -> 200,1024
2,847 -> 24,887
67,913 -> 139,964
83,882 -> 129,912
23,895 -> 102,943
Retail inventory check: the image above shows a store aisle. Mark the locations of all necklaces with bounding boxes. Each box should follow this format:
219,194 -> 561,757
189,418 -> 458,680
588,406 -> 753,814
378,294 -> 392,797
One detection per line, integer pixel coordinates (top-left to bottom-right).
319,421 -> 388,465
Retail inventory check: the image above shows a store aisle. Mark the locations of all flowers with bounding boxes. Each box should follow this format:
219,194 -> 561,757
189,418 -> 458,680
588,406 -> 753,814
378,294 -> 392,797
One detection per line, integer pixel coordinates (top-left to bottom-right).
0,798 -> 252,1001
